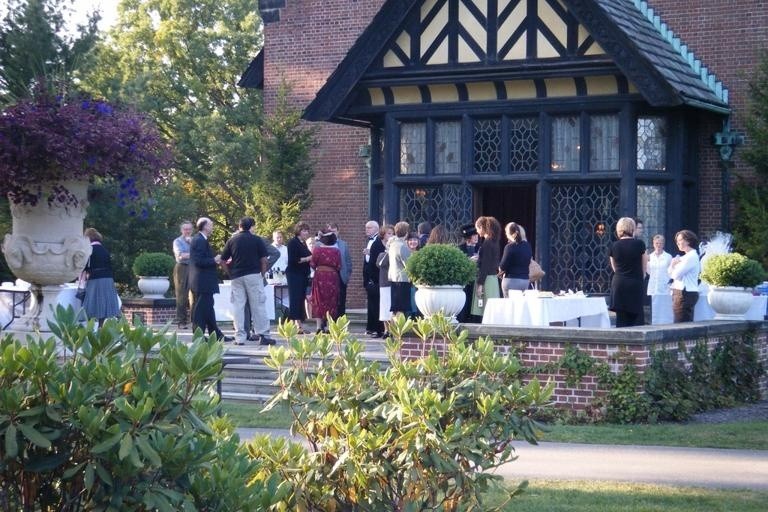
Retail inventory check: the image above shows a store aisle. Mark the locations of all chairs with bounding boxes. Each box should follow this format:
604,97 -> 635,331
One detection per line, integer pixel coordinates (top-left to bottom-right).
508,289 -> 539,298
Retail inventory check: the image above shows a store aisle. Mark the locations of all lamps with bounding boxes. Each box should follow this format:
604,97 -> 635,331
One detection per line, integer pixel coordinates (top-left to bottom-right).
358,144 -> 372,167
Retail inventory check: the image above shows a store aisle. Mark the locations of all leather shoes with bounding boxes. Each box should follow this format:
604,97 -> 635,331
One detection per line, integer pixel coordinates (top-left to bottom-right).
219,335 -> 234,342
178,322 -> 188,329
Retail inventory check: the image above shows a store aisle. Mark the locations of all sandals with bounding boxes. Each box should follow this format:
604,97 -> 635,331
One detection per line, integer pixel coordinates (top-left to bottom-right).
297,327 -> 331,335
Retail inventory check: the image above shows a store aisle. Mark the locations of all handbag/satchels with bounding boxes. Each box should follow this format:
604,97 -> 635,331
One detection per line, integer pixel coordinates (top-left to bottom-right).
375,251 -> 390,269
527,259 -> 545,283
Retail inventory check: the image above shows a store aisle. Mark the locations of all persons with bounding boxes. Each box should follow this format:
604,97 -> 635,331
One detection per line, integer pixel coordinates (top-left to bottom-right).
188,216 -> 234,342
668,230 -> 700,322
632,219 -> 643,238
647,235 -> 675,325
610,217 -> 647,327
220,217 -> 351,345
86,226 -> 121,328
362,214 -> 532,338
173,221 -> 195,328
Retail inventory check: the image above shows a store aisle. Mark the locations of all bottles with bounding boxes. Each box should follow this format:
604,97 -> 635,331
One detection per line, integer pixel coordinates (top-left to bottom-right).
477,294 -> 483,308
266,266 -> 283,283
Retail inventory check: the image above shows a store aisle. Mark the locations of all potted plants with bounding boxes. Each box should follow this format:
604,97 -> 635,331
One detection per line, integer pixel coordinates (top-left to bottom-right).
698,251 -> 766,321
403,243 -> 477,324
132,252 -> 176,300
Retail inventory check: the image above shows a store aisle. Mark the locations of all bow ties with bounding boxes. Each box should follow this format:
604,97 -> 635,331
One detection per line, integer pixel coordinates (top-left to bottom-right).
368,233 -> 379,241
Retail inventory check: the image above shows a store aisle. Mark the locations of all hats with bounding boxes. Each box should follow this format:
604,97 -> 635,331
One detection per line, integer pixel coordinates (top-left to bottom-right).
460,223 -> 478,238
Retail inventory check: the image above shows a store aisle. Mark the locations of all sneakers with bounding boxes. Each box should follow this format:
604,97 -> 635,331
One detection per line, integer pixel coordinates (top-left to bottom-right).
234,341 -> 245,346
260,337 -> 277,346
365,330 -> 392,340
